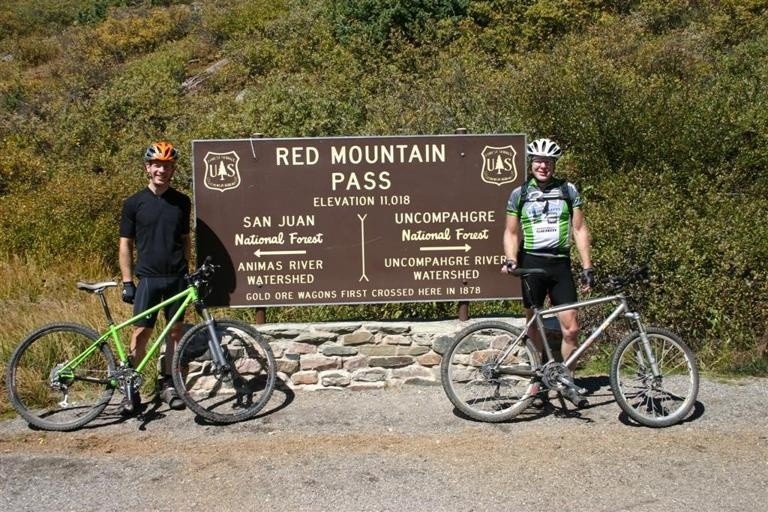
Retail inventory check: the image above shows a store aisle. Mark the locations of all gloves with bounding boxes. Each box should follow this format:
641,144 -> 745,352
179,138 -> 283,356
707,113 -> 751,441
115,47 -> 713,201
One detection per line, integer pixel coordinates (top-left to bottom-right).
499,260 -> 517,272
581,269 -> 596,288
122,281 -> 136,304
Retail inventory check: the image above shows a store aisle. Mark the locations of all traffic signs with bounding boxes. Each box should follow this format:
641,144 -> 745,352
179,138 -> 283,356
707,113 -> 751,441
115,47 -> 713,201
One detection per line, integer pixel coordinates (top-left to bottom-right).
192,133 -> 527,307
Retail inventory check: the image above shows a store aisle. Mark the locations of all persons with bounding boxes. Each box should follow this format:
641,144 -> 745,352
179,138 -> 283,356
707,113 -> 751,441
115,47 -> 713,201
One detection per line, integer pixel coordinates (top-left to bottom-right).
120,141 -> 192,410
503,138 -> 594,408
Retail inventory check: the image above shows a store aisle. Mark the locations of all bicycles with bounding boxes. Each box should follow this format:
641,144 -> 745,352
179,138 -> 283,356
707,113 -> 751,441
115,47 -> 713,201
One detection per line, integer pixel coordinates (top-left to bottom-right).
7,257 -> 277,431
441,267 -> 700,427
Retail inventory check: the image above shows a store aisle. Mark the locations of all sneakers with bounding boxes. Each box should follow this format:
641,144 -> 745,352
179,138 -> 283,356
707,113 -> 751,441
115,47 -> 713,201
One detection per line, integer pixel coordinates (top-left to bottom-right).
532,398 -> 544,408
119,391 -> 141,416
155,377 -> 186,410
561,385 -> 589,407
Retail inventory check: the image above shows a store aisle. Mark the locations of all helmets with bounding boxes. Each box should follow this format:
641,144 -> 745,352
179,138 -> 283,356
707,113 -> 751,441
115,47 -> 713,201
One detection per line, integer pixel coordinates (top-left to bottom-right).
527,139 -> 562,158
143,142 -> 179,163
